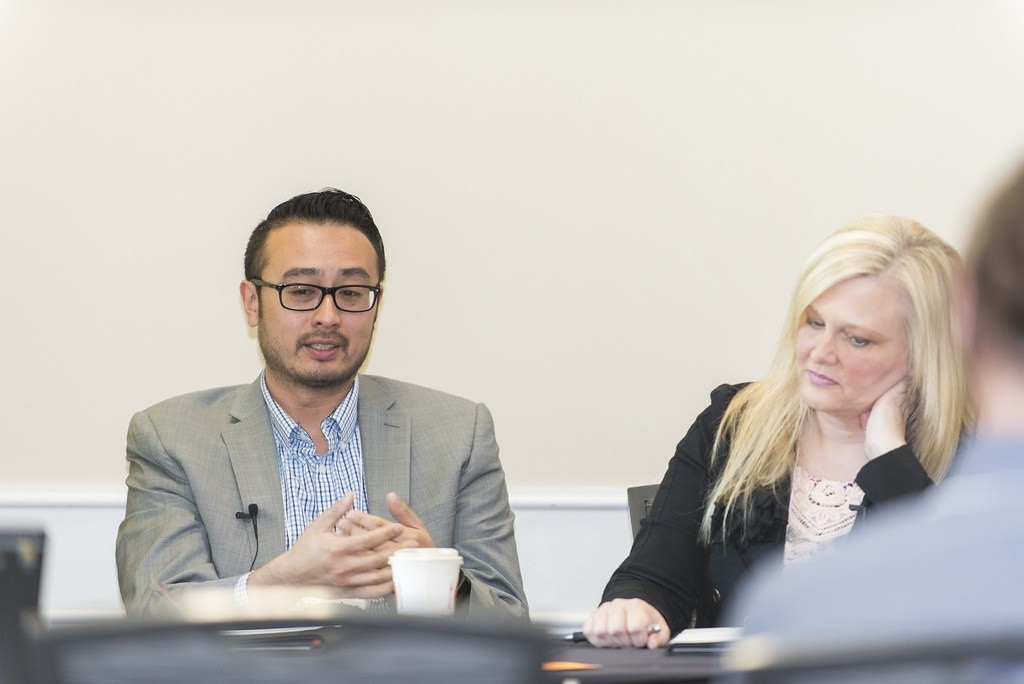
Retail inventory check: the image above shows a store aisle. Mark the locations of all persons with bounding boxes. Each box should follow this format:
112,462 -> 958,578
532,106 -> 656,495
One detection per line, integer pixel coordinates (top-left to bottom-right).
582,173 -> 1023,684
116,187 -> 534,625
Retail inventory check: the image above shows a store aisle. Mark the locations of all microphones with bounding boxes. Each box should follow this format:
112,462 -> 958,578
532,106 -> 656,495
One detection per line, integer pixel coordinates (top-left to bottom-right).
249,503 -> 258,530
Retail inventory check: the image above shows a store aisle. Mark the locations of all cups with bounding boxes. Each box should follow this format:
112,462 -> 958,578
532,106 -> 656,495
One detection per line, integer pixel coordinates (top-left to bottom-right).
387,547 -> 463,616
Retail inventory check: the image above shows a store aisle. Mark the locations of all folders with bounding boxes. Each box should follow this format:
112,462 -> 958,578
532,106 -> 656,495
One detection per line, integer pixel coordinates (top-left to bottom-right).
214,625 -> 357,656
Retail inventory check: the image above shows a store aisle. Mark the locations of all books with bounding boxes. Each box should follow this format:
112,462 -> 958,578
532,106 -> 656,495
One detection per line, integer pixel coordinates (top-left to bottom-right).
669,627 -> 739,656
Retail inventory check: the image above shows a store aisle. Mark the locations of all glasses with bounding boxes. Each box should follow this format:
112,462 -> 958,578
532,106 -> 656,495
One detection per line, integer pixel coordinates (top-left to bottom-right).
252,278 -> 382,311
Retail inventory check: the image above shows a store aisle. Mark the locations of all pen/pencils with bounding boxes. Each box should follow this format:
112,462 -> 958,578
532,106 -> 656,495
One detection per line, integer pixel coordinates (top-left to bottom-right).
561,623 -> 661,643
